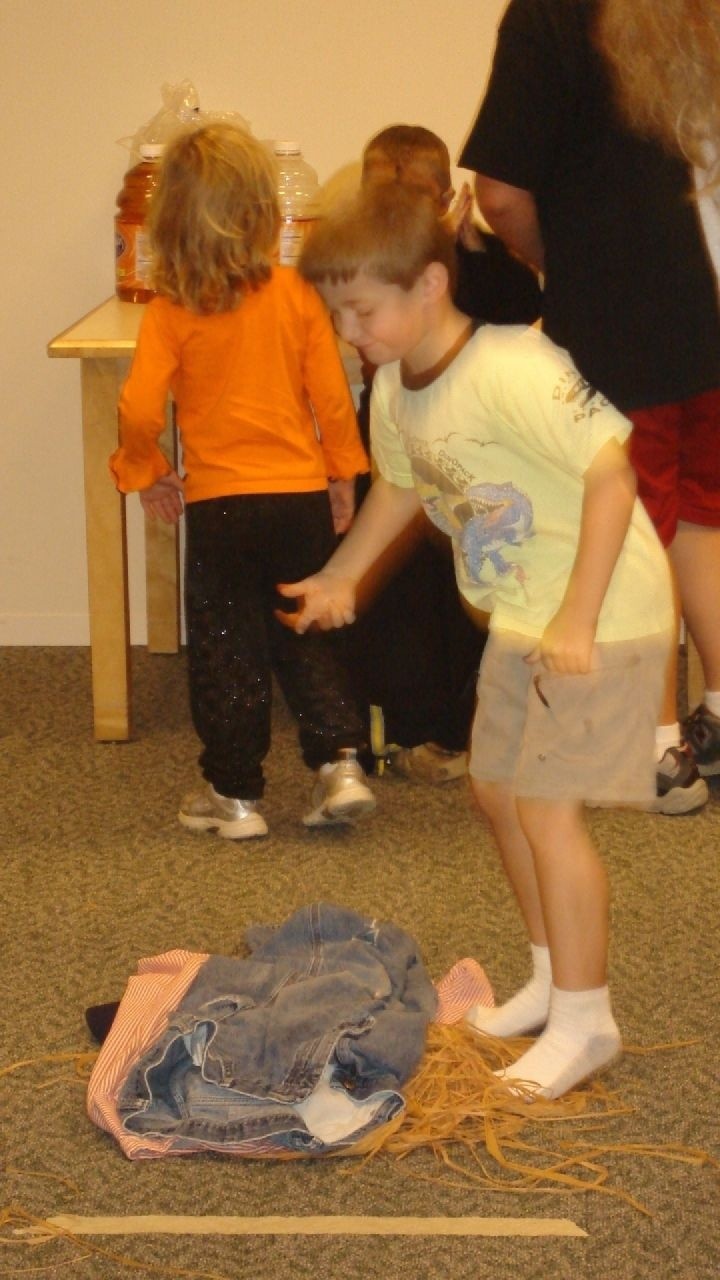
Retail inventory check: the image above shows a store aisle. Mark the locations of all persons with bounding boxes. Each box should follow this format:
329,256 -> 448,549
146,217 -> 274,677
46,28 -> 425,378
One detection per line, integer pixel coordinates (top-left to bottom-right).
107,120 -> 379,843
341,126 -> 546,784
458,0 -> 720,815
596,1 -> 720,296
278,178 -> 679,1105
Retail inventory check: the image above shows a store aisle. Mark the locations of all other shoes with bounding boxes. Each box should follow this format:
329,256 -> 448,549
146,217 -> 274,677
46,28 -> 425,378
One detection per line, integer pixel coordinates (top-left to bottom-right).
395,741 -> 471,781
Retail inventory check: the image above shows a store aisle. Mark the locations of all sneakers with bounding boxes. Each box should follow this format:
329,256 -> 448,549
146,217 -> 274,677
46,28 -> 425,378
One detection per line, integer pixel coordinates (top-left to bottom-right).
178,785 -> 268,838
678,704 -> 720,775
302,759 -> 377,825
584,742 -> 710,812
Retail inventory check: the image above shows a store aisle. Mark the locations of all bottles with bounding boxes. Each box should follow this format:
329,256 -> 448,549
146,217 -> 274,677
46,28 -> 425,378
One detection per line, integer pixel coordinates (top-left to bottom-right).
114,144 -> 164,304
272,141 -> 318,266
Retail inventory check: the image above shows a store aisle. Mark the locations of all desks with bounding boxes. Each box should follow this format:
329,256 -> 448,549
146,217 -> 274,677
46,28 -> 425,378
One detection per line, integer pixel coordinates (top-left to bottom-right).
48,291 -> 544,740
